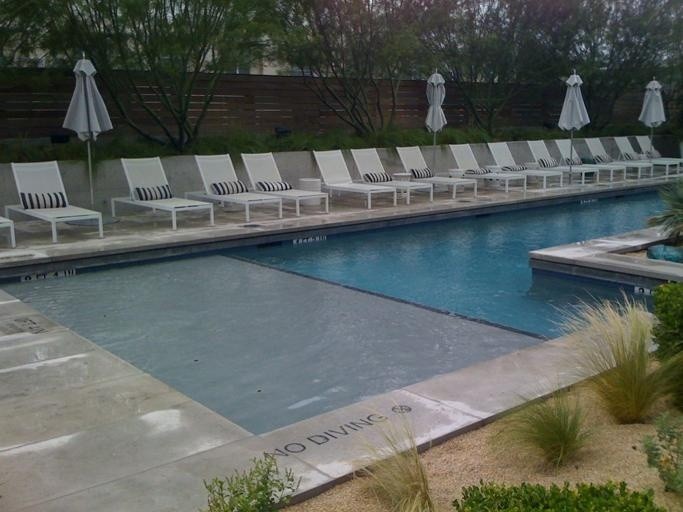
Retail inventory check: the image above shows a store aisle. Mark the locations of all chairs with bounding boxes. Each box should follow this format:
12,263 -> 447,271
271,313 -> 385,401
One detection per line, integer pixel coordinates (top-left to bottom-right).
396,145 -> 477,198
487,142 -> 564,189
636,135 -> 682,174
615,137 -> 679,176
527,140 -> 600,183
350,148 -> 434,205
181,152 -> 283,223
585,138 -> 653,178
3,160 -> 103,245
556,139 -> 627,182
449,143 -> 528,193
313,149 -> 397,209
239,150 -> 328,216
109,154 -> 215,231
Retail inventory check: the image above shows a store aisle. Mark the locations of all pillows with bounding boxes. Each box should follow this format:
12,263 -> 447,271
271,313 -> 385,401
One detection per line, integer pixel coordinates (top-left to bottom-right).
646,150 -> 661,159
18,190 -> 66,210
593,154 -> 613,165
411,166 -> 434,179
567,157 -> 582,165
465,167 -> 493,175
362,172 -> 392,182
624,152 -> 642,160
500,166 -> 528,171
133,181 -> 173,202
257,179 -> 290,193
539,158 -> 558,167
210,177 -> 248,197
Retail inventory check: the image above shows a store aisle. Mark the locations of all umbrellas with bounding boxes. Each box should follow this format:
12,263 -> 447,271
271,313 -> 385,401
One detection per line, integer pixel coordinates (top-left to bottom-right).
410,68 -> 451,195
61,52 -> 119,227
637,75 -> 666,179
554,69 -> 592,186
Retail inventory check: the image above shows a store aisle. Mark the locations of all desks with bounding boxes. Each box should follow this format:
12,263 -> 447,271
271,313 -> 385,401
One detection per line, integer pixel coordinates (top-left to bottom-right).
296,176 -> 322,208
